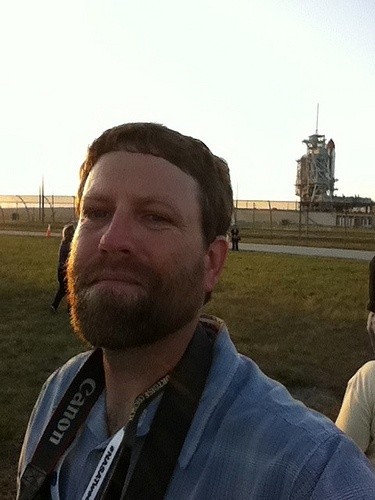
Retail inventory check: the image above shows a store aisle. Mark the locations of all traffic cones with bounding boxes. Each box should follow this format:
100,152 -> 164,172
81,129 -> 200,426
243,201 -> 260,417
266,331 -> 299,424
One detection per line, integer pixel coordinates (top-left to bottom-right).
46,229 -> 51,237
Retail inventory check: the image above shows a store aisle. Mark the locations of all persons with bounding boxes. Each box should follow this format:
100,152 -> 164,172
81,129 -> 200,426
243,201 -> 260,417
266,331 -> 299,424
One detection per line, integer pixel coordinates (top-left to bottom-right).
13,122 -> 375,500
229,224 -> 241,252
48,223 -> 74,315
330,255 -> 375,472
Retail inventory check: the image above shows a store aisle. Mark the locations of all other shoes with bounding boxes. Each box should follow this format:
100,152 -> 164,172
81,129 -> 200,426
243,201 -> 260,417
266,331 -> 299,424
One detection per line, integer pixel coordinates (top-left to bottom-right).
50,304 -> 57,316
